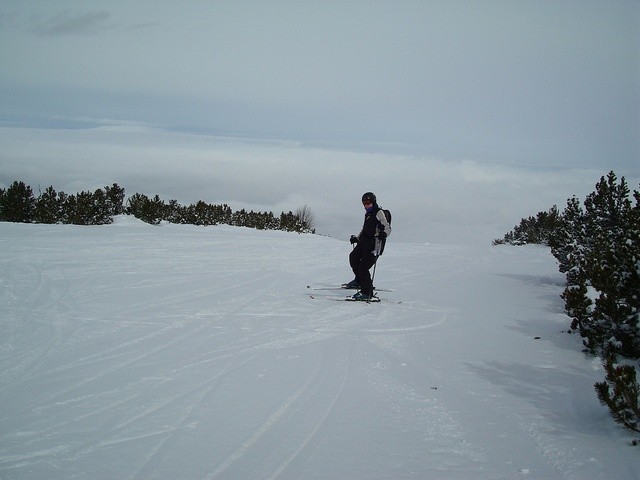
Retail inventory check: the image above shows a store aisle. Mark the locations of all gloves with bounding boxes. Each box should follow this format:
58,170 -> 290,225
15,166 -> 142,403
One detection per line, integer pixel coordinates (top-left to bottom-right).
350,235 -> 358,244
378,231 -> 387,240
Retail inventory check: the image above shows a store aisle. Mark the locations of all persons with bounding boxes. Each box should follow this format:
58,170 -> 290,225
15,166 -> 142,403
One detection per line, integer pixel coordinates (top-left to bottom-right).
342,192 -> 391,299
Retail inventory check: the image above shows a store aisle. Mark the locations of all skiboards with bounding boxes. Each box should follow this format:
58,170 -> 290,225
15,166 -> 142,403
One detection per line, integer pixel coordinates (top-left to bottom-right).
304,283 -> 402,303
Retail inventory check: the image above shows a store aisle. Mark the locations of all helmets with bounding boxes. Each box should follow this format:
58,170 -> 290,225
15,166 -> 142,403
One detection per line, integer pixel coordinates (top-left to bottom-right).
362,192 -> 376,204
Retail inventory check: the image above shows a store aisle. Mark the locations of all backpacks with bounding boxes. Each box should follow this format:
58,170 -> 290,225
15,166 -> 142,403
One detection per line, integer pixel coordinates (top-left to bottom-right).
376,208 -> 391,231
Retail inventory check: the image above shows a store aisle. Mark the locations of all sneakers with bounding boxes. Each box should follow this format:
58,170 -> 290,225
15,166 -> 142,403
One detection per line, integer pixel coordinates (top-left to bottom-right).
346,279 -> 361,289
352,290 -> 373,299
